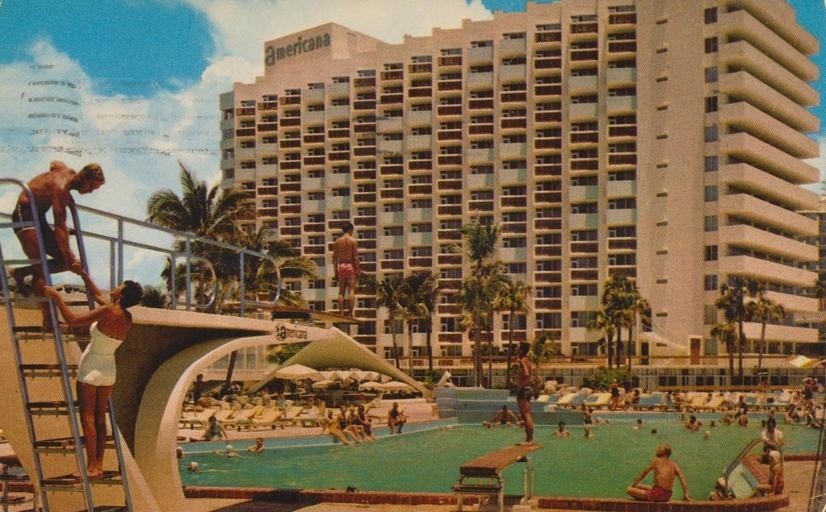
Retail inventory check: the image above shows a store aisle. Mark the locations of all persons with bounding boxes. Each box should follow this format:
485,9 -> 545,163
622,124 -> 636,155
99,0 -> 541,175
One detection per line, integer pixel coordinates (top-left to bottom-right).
8,159 -> 106,296
177,374 -> 404,471
42,268 -> 143,481
516,343 -> 534,445
332,222 -> 362,321
480,404 -> 524,426
557,379 -> 825,501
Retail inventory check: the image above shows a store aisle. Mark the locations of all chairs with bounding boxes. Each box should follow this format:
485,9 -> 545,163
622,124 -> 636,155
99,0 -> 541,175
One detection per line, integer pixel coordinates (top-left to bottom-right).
534,387 -> 802,412
179,403 -> 360,428
